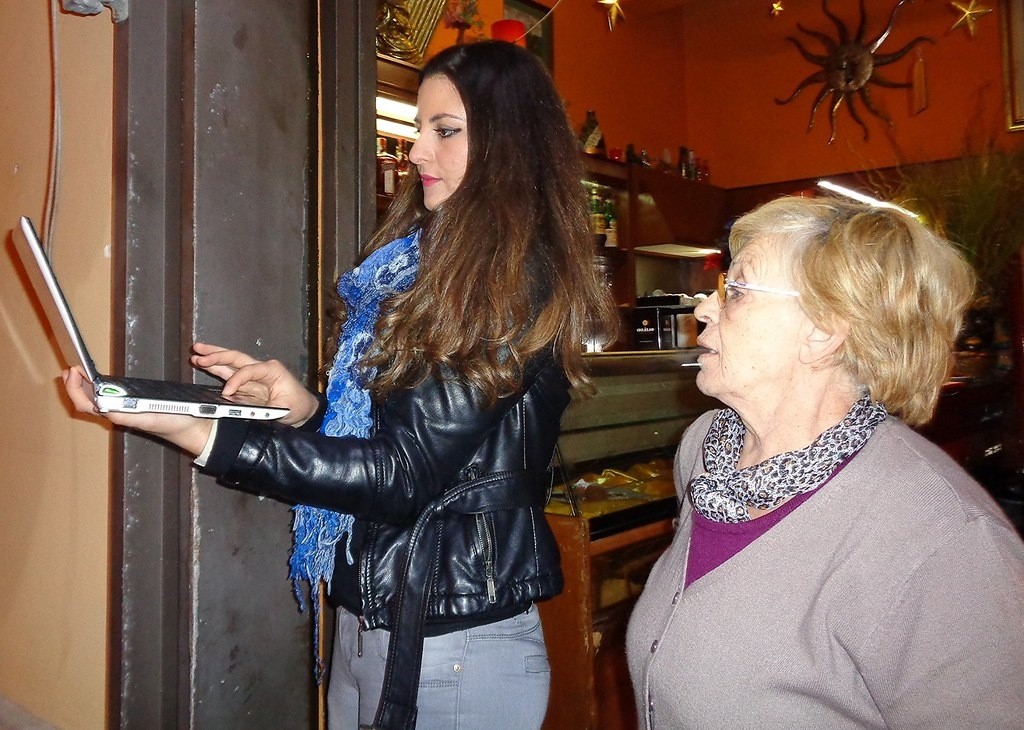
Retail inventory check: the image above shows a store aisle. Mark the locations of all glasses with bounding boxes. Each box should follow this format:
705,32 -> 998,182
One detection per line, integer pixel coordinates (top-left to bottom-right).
718,272 -> 800,311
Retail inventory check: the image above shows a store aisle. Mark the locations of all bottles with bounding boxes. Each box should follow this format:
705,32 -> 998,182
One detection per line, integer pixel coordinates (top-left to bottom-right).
686,150 -> 697,180
397,138 -> 409,181
376,138 -> 399,195
580,110 -> 606,156
679,146 -> 687,177
590,187 -> 607,245
604,200 -> 617,247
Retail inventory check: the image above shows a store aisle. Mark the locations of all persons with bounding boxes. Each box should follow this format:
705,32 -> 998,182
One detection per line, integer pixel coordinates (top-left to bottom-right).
625,195 -> 1024,730
62,40 -> 621,730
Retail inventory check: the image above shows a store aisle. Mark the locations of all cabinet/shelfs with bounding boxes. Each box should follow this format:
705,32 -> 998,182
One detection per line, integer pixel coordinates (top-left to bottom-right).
572,155 -> 728,352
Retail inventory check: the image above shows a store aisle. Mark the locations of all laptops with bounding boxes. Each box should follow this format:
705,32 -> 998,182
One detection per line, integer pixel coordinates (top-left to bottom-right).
11,215 -> 291,419
